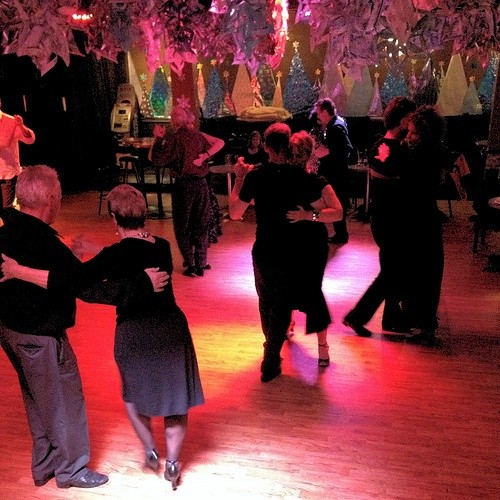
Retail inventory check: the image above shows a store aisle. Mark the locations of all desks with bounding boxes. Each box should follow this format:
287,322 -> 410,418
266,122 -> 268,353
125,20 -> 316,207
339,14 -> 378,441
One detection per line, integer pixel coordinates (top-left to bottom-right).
110,137 -> 172,219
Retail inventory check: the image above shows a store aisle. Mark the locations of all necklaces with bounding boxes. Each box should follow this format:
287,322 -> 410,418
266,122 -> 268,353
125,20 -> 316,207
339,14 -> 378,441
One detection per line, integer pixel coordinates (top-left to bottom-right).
135,232 -> 150,238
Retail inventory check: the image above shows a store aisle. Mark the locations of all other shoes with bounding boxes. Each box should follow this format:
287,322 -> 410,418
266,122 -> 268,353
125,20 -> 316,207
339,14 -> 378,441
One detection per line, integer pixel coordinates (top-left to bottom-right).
329,232 -> 349,244
344,314 -> 371,337
56,467 -> 108,488
384,333 -> 404,342
262,367 -> 281,383
406,333 -> 443,348
35,469 -> 55,487
194,267 -> 204,276
183,265 -> 194,276
200,264 -> 210,269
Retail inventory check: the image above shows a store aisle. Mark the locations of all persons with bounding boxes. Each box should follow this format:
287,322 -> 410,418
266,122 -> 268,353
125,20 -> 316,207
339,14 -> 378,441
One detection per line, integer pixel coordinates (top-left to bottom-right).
0,101 -> 35,208
0,165 -> 171,489
229,123 -> 342,382
342,97 -> 450,342
308,99 -> 354,245
148,109 -> 225,276
0,183 -> 205,491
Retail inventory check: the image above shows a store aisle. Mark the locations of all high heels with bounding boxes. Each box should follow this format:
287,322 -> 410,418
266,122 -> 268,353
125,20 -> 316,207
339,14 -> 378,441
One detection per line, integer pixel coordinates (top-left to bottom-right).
147,449 -> 160,471
164,460 -> 182,491
317,343 -> 330,366
287,319 -> 295,340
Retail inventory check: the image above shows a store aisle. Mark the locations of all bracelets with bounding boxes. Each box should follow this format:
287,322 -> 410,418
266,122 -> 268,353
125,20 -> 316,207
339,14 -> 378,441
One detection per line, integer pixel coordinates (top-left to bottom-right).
206,152 -> 210,156
313,211 -> 320,222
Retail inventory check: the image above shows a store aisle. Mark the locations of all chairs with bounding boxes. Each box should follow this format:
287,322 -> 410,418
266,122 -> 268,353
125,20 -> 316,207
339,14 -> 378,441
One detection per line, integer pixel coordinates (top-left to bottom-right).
99,156 -> 148,216
